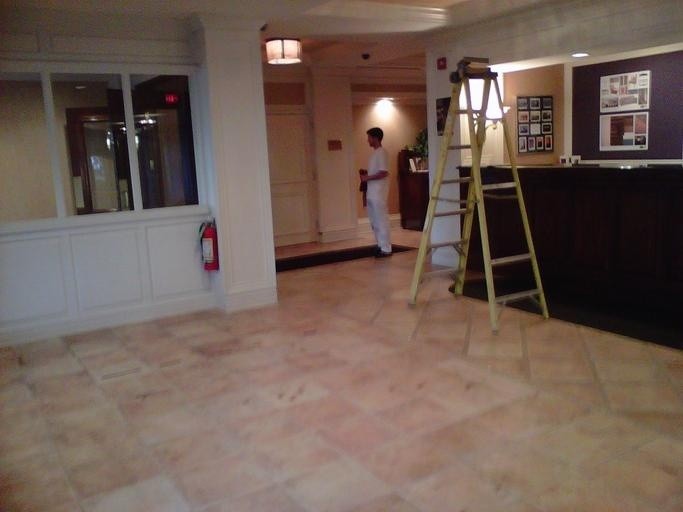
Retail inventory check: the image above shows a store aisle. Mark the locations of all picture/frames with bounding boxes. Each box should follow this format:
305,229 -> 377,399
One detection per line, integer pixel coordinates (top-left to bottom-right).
517,95 -> 553,154
599,69 -> 651,152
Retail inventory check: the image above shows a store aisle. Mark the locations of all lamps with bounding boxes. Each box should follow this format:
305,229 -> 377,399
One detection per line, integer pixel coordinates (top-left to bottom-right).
263,37 -> 303,66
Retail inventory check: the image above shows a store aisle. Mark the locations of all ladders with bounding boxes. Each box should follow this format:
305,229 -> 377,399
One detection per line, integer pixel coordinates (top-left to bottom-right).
407,70 -> 549,336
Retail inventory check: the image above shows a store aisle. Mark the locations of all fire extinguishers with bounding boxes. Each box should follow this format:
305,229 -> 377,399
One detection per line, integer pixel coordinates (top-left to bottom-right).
199,217 -> 219,271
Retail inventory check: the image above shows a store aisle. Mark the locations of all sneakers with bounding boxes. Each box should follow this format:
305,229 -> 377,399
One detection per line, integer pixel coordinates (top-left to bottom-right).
374,247 -> 392,257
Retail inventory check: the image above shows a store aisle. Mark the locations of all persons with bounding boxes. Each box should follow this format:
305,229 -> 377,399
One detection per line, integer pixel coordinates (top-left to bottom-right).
359,127 -> 393,259
